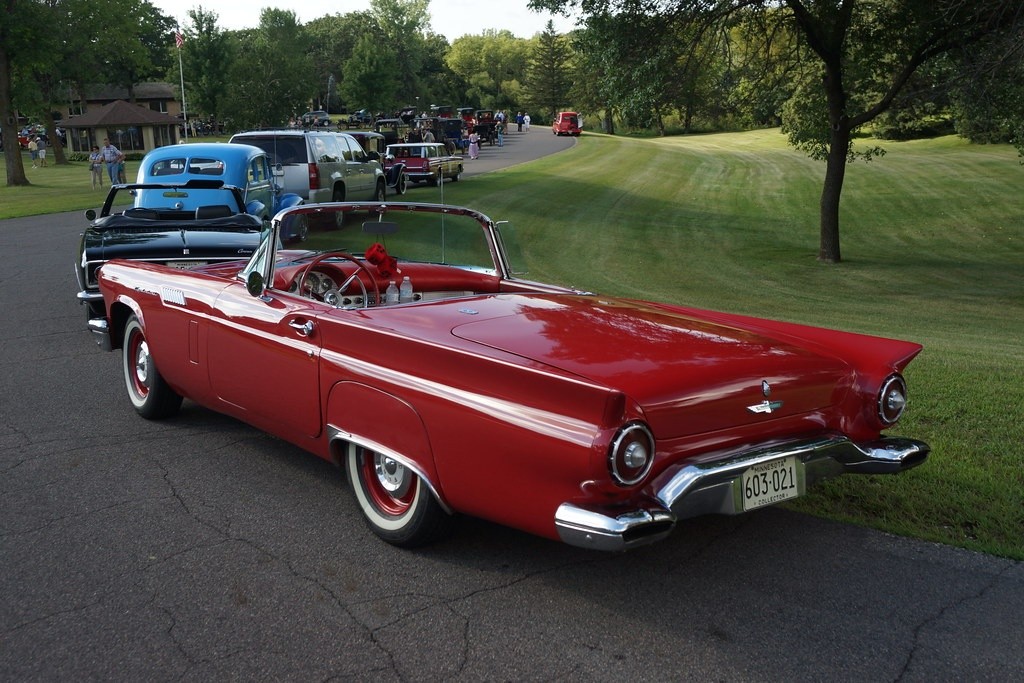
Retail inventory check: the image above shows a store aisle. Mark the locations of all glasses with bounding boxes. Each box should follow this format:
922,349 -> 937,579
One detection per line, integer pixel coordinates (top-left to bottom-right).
93,149 -> 97,150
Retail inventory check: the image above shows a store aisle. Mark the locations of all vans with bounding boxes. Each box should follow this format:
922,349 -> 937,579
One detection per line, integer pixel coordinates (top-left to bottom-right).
553,111 -> 583,136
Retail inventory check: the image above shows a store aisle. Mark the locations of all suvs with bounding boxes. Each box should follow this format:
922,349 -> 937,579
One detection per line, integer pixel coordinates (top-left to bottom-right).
227,129 -> 387,229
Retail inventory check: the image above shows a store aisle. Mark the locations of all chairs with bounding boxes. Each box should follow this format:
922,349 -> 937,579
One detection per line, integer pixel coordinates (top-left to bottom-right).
195,204 -> 232,220
122,209 -> 160,219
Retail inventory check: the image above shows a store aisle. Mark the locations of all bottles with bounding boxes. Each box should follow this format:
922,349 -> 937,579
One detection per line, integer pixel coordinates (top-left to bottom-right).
400,276 -> 414,304
386,281 -> 399,306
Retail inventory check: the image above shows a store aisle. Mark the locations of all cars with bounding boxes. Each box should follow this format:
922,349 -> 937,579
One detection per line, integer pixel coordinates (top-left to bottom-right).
0,120 -> 68,149
130,139 -> 308,242
88,200 -> 930,547
178,112 -> 228,137
72,179 -> 284,332
299,105 -> 507,152
384,142 -> 465,187
346,131 -> 408,194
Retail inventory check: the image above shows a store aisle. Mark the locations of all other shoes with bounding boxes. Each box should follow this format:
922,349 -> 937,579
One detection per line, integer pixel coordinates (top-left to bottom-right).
32,165 -> 38,168
93,186 -> 95,190
100,185 -> 102,190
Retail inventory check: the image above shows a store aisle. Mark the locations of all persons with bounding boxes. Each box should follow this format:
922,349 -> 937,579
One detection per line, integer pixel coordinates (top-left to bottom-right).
185,114 -> 319,138
347,108 -> 531,160
20,123 -> 48,169
88,138 -> 127,191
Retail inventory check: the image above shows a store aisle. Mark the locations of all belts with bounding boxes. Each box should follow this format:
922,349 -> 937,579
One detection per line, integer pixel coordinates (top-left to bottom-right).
106,161 -> 113,163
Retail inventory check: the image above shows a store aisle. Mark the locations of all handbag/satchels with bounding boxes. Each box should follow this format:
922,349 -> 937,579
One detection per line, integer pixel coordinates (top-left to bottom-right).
89,164 -> 94,170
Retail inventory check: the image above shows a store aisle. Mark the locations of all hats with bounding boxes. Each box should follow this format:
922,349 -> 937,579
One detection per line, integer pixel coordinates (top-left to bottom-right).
36,137 -> 41,141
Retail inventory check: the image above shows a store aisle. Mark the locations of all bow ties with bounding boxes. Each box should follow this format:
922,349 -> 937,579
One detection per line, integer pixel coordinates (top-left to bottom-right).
106,145 -> 110,148
